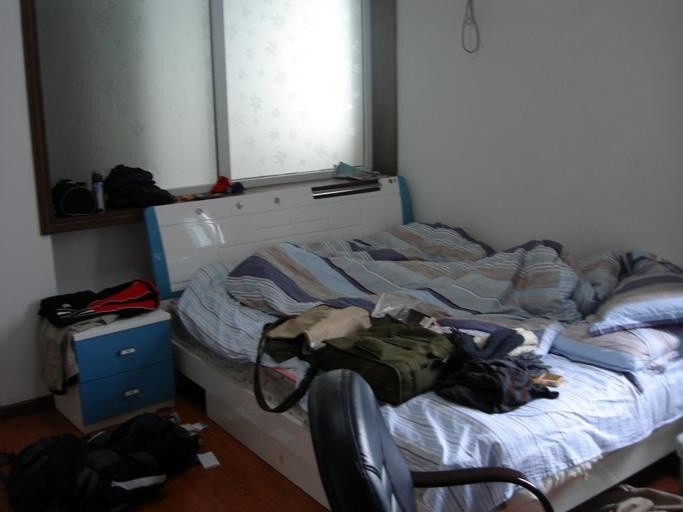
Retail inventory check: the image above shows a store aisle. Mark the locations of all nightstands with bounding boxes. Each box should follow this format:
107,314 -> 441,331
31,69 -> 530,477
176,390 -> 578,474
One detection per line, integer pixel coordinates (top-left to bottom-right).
52,307 -> 176,436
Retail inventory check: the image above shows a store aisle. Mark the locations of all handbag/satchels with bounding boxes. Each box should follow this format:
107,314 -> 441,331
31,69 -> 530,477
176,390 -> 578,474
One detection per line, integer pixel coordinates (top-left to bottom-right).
265,305 -> 372,354
322,314 -> 452,405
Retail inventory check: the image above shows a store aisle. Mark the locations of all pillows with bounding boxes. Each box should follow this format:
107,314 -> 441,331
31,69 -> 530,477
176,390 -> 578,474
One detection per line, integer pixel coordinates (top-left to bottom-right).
549,259 -> 683,377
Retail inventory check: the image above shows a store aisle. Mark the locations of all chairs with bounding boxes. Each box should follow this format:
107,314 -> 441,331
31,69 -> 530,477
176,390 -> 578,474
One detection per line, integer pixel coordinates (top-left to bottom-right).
307,367 -> 552,510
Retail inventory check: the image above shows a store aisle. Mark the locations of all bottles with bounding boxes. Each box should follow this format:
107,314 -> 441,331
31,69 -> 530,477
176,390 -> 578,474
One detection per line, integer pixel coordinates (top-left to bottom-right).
91,171 -> 106,211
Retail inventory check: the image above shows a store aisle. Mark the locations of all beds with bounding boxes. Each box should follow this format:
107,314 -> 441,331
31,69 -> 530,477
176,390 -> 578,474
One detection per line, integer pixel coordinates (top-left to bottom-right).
142,175 -> 683,510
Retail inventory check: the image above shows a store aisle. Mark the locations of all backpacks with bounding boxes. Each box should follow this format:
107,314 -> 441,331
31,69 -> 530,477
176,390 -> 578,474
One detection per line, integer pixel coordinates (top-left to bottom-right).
0,412 -> 200,512
